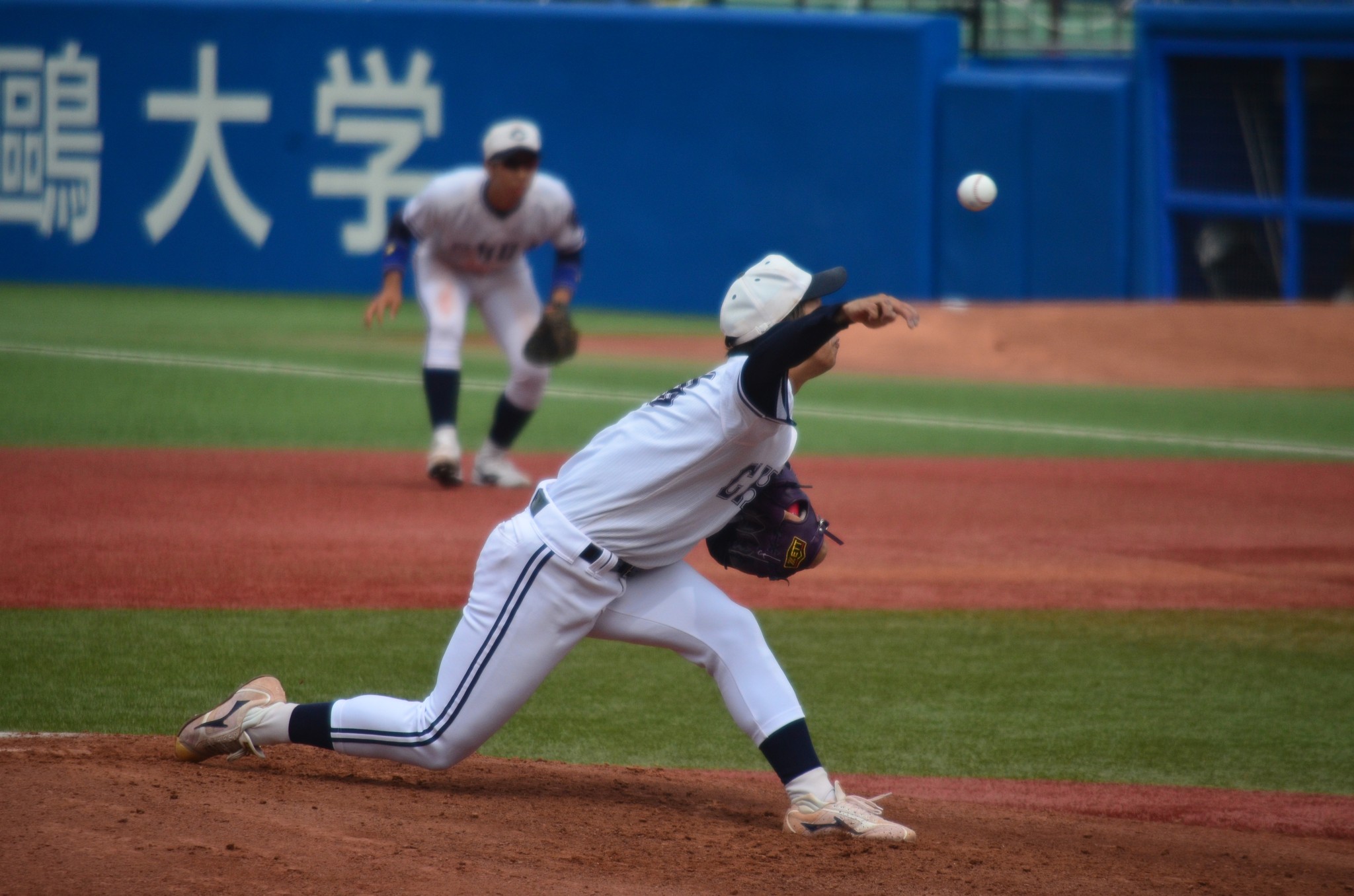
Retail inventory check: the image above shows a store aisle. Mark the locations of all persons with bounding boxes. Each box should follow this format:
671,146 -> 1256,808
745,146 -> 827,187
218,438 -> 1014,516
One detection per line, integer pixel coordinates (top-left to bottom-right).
366,120 -> 584,487
178,252 -> 920,839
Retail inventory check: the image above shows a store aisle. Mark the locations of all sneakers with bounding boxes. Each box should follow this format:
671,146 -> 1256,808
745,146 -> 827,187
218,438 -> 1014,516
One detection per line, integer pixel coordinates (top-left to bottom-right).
426,435 -> 466,487
470,454 -> 532,489
175,677 -> 290,763
781,784 -> 915,841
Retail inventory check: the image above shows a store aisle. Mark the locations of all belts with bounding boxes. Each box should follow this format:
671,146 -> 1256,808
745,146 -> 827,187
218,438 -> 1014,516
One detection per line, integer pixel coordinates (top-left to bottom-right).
529,492 -> 638,579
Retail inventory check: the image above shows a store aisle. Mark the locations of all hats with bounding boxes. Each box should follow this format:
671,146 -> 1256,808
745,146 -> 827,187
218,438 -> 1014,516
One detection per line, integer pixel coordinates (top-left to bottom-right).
482,119 -> 543,167
719,254 -> 849,351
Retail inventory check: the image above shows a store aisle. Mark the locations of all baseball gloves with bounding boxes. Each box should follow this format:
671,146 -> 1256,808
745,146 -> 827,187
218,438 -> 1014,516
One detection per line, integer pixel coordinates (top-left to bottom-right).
707,459 -> 831,578
521,305 -> 577,367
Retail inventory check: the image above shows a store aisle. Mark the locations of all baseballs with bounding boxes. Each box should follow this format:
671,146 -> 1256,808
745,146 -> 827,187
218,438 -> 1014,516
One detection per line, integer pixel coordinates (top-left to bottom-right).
957,172 -> 998,210
